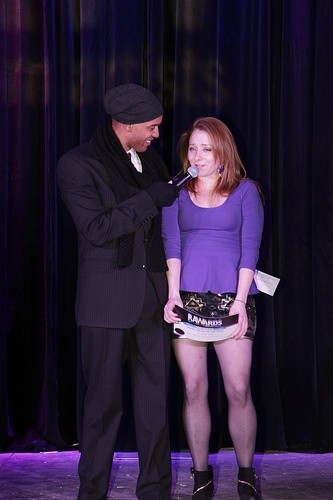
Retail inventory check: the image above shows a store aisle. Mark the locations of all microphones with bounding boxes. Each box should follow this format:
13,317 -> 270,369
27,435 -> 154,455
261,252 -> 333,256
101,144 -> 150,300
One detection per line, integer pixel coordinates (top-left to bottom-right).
168,166 -> 199,191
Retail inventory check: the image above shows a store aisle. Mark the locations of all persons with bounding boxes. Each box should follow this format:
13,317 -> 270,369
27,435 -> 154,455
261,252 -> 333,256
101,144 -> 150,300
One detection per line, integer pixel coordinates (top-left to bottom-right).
161,116 -> 281,500
56,83 -> 199,500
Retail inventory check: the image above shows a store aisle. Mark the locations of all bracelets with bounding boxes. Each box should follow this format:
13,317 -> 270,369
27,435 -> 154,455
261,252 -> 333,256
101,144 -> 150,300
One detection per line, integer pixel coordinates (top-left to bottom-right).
234,299 -> 247,306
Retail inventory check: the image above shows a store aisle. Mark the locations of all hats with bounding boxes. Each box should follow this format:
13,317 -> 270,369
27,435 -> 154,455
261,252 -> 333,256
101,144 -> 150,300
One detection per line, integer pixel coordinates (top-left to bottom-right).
102,84 -> 164,125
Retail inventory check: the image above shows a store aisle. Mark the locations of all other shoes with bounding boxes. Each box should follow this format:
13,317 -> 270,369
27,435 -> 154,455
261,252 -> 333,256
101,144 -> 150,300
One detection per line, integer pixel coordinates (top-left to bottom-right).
238,466 -> 259,500
191,465 -> 216,500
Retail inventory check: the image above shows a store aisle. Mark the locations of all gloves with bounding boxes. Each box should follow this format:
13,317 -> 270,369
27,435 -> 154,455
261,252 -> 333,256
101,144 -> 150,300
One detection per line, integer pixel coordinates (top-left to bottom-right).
149,179 -> 181,207
